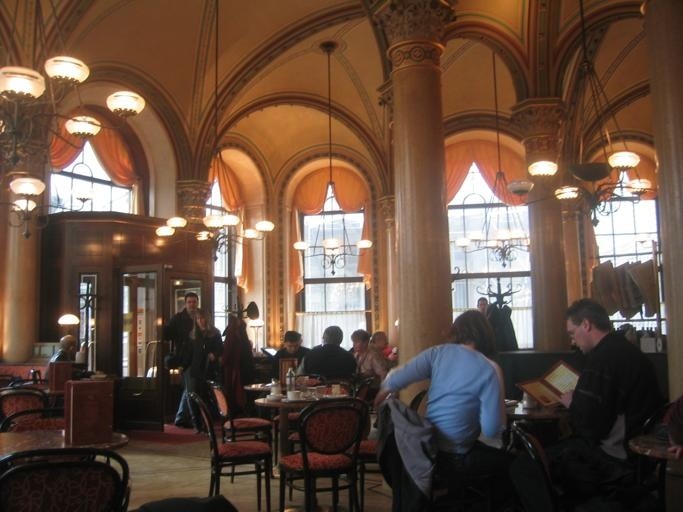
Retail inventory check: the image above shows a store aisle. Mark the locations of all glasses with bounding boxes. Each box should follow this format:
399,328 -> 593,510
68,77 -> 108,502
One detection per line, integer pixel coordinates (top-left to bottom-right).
566,321 -> 589,340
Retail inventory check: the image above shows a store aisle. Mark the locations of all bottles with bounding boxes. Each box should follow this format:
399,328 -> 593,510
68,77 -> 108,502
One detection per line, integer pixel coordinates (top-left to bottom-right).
285,367 -> 295,393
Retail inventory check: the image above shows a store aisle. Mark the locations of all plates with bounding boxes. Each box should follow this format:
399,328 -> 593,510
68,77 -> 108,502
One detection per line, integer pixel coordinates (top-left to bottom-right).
506,400 -> 519,407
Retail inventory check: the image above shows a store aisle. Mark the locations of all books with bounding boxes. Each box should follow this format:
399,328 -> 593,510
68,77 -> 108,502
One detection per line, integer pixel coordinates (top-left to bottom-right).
514,359 -> 581,407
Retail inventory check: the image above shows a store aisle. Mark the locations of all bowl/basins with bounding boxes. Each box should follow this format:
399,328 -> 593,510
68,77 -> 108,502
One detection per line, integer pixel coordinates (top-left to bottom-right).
267,395 -> 284,401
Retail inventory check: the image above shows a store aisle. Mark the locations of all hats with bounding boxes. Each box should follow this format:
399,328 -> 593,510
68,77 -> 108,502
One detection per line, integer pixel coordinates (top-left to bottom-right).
285,331 -> 300,341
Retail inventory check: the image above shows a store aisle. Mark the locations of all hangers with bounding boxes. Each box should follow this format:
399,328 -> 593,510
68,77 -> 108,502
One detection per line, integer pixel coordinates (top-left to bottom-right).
225,303 -> 248,319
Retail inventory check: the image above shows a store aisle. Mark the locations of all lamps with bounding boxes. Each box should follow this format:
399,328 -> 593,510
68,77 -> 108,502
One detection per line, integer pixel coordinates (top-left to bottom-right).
2,2 -> 146,165
249,319 -> 264,351
58,314 -> 80,336
455,54 -> 531,268
156,0 -> 274,260
3,163 -> 95,239
294,43 -> 373,276
508,2 -> 651,226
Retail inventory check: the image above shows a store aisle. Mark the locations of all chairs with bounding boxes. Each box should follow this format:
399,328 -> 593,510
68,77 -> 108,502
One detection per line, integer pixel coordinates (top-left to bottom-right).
207,380 -> 272,484
0,409 -> 68,431
278,398 -> 370,512
628,400 -> 683,512
359,388 -> 429,510
3,368 -> 70,395
190,392 -> 273,512
0,447 -> 130,510
1,388 -> 49,418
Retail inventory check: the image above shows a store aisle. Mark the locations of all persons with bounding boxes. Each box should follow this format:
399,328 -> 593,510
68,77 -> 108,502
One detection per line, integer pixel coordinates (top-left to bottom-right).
350,329 -> 385,400
165,309 -> 222,433
44,335 -> 84,385
162,292 -> 199,370
80,342 -> 87,352
545,299 -> 661,487
370,330 -> 388,352
373,310 -> 520,482
273,331 -> 311,372
667,397 -> 682,460
477,298 -> 487,313
297,326 -> 357,380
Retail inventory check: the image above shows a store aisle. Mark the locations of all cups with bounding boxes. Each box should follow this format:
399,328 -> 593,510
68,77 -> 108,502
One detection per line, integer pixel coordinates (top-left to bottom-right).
333,385 -> 341,396
524,392 -> 540,411
288,391 -> 301,401
